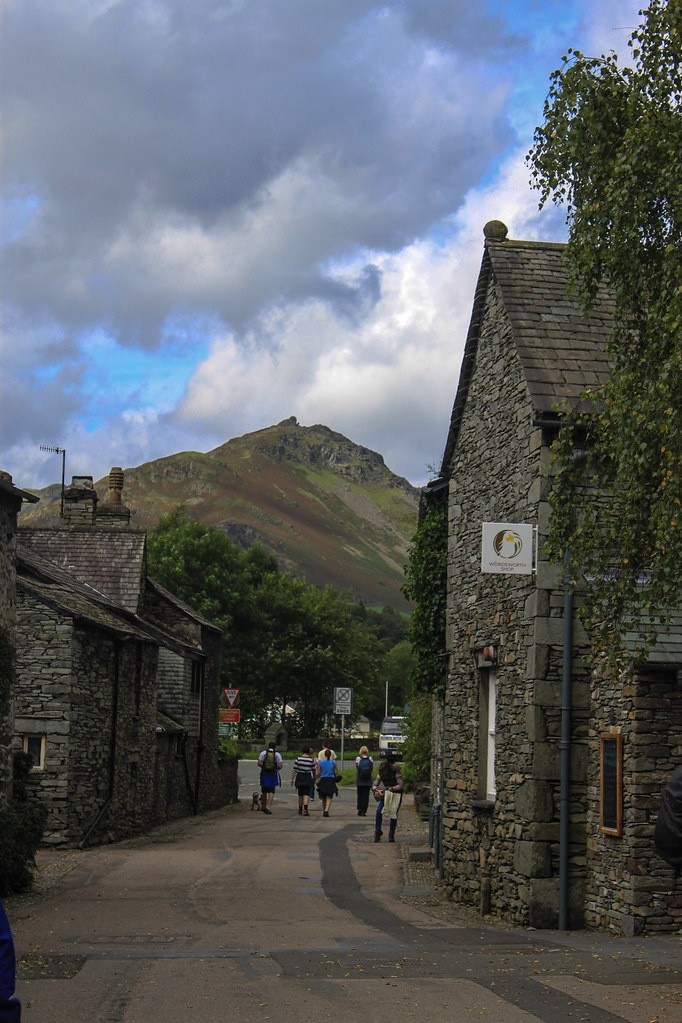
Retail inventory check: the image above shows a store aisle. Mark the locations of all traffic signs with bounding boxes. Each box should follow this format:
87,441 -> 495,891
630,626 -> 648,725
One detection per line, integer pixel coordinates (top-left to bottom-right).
219,707 -> 240,723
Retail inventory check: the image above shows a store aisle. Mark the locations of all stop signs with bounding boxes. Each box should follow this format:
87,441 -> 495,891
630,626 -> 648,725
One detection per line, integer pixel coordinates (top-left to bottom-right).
334,687 -> 352,715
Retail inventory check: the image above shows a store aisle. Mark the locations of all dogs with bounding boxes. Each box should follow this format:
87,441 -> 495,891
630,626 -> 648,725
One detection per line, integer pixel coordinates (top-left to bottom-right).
251,792 -> 263,811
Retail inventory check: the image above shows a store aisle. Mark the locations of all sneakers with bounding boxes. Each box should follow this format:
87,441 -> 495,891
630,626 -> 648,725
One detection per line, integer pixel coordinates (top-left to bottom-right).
303,809 -> 310,816
261,806 -> 267,813
388,835 -> 395,842
298,810 -> 303,816
361,812 -> 367,816
374,830 -> 384,843
323,811 -> 329,817
264,807 -> 272,814
358,811 -> 362,815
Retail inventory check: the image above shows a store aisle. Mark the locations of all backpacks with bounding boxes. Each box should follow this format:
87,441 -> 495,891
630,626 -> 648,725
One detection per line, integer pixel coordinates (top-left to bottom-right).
357,754 -> 372,780
262,749 -> 277,771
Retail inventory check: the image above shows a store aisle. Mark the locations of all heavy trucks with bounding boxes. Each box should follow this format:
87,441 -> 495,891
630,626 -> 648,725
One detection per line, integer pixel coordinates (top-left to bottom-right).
378,712 -> 417,763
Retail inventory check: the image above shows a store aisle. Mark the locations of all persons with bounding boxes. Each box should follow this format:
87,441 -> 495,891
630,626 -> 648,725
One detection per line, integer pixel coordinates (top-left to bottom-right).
257,742 -> 283,815
372,761 -> 404,843
308,748 -> 320,801
290,745 -> 316,816
318,741 -> 338,761
356,746 -> 373,817
317,749 -> 339,817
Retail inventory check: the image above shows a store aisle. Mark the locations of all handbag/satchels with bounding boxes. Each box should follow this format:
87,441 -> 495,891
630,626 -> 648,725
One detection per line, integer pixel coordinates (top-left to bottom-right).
380,789 -> 401,820
